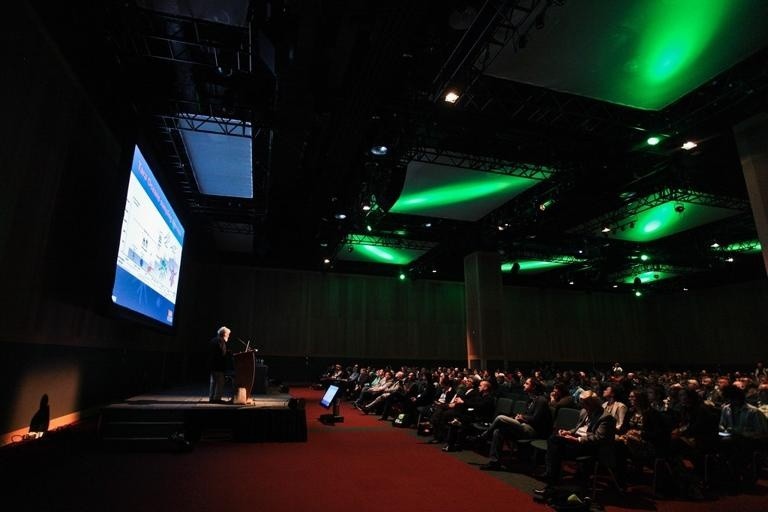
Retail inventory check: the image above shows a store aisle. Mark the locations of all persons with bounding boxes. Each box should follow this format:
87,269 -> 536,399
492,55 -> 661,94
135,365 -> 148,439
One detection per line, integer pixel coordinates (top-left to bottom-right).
209,327 -> 231,403
320,355 -> 768,501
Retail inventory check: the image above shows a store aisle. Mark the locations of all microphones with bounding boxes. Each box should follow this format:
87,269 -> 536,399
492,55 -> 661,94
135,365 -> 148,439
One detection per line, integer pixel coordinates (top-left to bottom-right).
239,340 -> 246,350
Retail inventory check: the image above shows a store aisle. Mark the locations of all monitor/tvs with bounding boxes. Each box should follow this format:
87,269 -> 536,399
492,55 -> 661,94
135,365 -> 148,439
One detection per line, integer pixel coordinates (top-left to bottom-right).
321,385 -> 339,406
245,340 -> 250,352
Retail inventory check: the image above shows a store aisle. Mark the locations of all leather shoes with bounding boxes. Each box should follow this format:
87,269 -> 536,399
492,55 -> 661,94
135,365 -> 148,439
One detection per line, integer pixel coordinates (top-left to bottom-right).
351,399 -> 565,498
